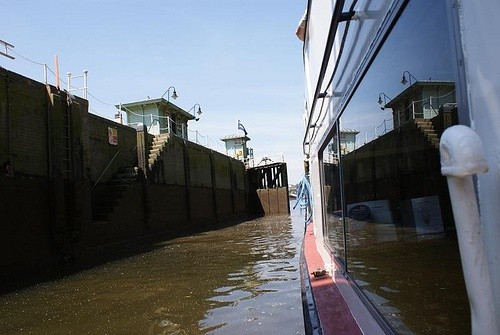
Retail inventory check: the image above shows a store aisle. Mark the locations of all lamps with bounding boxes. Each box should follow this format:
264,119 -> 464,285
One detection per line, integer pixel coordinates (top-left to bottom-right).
188,104 -> 202,116
162,86 -> 177,102
402,70 -> 416,86
378,92 -> 391,110
